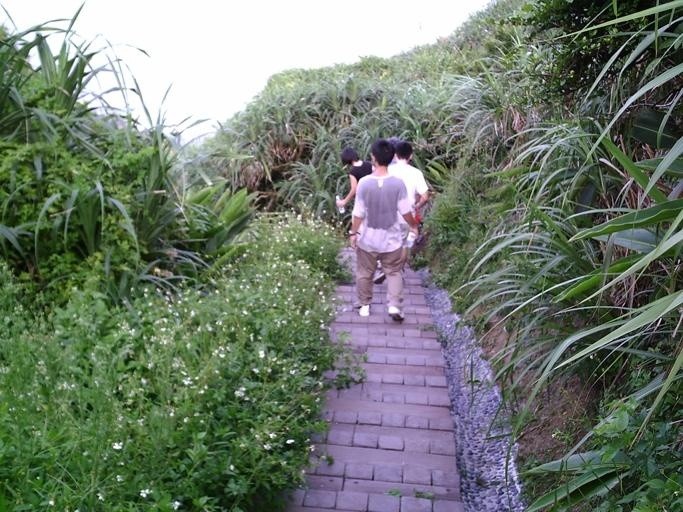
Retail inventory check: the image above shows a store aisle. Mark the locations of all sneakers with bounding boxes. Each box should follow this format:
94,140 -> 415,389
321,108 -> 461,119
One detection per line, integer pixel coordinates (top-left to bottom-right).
373,269 -> 386,284
358,305 -> 370,317
388,306 -> 404,321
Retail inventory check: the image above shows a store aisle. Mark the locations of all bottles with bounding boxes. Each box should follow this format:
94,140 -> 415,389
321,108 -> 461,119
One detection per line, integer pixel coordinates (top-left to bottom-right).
406,229 -> 416,247
335,196 -> 345,213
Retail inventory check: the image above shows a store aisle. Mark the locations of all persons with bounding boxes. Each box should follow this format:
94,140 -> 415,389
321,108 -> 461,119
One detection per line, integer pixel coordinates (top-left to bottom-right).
336,137 -> 431,322
